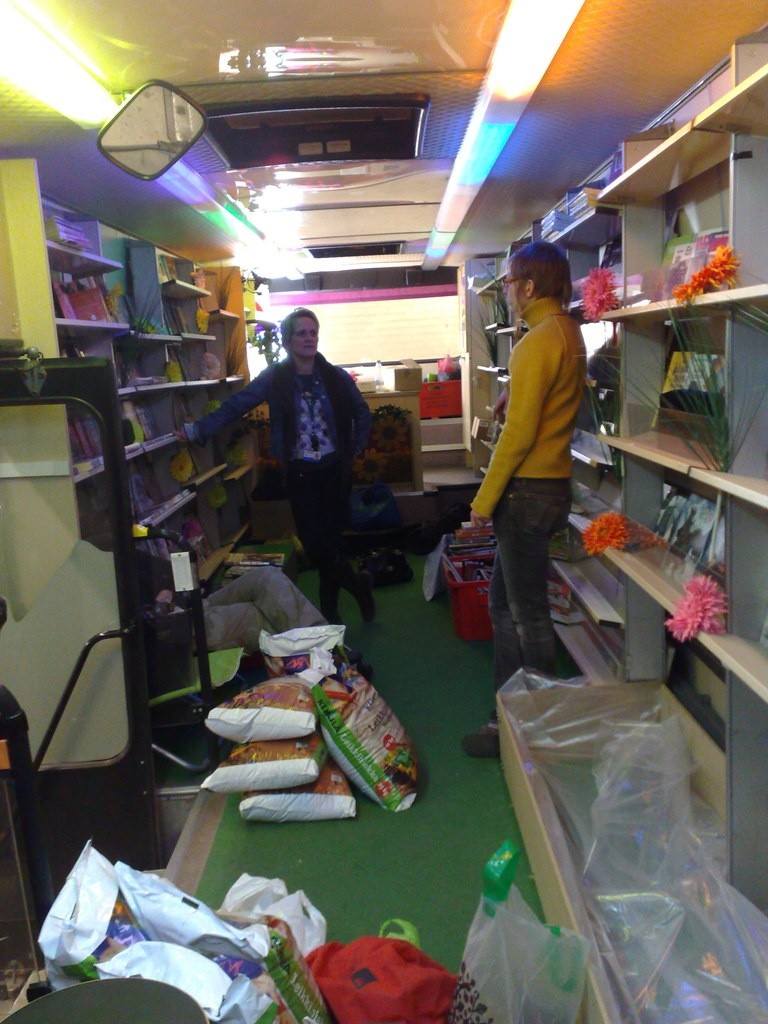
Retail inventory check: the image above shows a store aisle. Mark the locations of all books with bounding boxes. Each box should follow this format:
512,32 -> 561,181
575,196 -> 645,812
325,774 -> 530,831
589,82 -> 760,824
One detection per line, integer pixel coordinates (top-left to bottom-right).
68,416 -> 102,464
168,342 -> 208,382
51,269 -> 111,322
540,150 -> 622,241
651,352 -> 727,430
172,396 -> 192,431
652,485 -> 725,588
46,216 -> 94,252
136,522 -> 173,561
446,521 -> 497,556
223,552 -> 285,579
158,254 -> 178,282
653,227 -> 728,302
162,294 -> 199,335
122,400 -> 160,441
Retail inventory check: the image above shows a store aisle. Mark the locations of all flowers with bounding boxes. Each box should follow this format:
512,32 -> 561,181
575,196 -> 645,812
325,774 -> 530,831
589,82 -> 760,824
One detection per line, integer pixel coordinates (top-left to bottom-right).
580,265 -> 622,324
583,509 -> 632,557
674,244 -> 741,306
665,573 -> 727,644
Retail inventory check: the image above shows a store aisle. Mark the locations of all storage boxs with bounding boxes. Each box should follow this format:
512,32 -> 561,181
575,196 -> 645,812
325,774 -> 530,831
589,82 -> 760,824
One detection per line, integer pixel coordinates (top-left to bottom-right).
419,379 -> 463,419
384,366 -> 421,392
248,496 -> 299,541
442,552 -> 493,642
657,405 -> 719,443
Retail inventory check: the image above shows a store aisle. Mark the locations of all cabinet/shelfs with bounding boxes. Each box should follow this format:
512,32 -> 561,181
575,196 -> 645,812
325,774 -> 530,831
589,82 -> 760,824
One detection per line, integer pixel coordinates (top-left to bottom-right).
418,416 -> 485,488
37,195 -> 253,611
470,23 -> 768,922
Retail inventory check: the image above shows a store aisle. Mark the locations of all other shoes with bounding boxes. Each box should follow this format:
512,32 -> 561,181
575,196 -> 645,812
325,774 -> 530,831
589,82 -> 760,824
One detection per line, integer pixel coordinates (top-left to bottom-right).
359,663 -> 373,681
353,568 -> 377,622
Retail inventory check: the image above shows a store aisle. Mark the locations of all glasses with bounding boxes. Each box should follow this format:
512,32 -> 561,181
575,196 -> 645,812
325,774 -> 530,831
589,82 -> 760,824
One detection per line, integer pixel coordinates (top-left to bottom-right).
502,278 -> 519,285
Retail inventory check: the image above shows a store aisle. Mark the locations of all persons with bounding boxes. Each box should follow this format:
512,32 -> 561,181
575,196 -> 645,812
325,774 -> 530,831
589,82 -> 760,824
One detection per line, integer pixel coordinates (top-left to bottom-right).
81,517 -> 373,682
171,310 -> 375,625
315,454 -> 319,459
470,241 -> 588,734
663,245 -> 692,300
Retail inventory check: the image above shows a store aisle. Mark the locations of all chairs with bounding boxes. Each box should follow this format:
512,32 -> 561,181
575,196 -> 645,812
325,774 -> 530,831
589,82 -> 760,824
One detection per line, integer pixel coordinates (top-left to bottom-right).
147,643 -> 246,772
